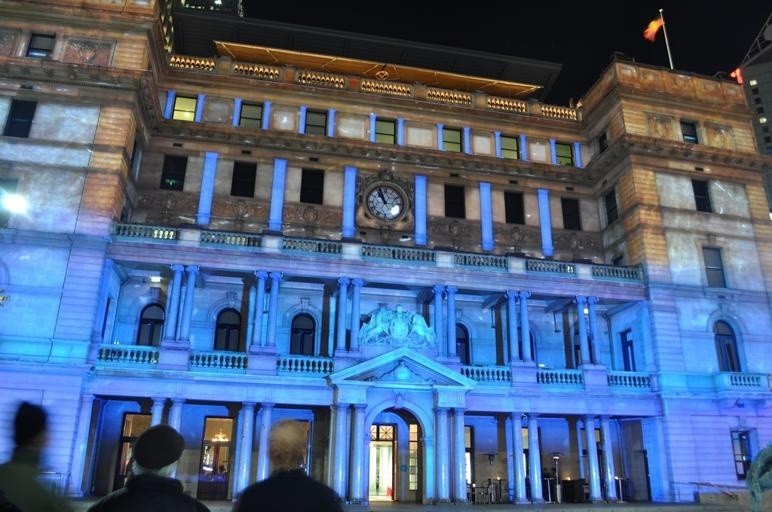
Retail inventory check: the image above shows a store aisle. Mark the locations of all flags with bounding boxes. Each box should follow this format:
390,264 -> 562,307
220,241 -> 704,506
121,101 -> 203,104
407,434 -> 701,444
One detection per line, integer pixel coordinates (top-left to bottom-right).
644,17 -> 665,40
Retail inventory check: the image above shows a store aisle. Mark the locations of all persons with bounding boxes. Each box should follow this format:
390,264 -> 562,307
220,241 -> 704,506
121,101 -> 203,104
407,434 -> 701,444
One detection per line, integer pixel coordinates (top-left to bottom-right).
552,467 -> 561,505
86,423 -> 210,511
232,419 -> 345,512
0,402 -> 72,510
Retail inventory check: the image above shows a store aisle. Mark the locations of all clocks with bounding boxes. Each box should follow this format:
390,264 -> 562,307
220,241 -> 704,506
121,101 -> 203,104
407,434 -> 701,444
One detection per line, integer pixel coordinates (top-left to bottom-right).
367,186 -> 405,221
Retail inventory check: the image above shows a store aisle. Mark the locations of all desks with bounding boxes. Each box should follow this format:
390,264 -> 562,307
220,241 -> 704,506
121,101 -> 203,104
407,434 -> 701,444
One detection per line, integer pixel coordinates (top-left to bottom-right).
472,487 -> 491,505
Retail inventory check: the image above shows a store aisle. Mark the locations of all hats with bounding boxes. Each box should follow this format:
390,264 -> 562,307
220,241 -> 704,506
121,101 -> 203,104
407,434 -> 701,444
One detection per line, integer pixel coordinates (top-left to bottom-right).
134,425 -> 185,469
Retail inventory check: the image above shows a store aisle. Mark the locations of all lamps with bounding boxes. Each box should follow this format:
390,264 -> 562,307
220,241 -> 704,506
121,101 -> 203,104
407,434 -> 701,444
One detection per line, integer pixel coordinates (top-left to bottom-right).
211,422 -> 228,441
